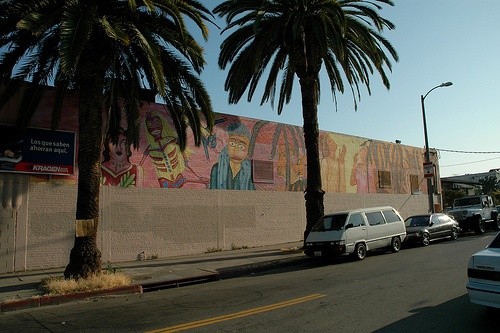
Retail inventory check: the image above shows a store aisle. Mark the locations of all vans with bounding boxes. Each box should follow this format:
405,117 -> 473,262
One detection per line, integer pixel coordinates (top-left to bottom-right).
303,206 -> 407,261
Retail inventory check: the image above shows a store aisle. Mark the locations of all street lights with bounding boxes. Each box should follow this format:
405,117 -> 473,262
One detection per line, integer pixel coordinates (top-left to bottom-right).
420,81 -> 453,214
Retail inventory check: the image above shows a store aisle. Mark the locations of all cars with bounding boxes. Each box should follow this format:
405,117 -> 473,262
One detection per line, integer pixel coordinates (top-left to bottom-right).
403,212 -> 460,246
465,231 -> 500,309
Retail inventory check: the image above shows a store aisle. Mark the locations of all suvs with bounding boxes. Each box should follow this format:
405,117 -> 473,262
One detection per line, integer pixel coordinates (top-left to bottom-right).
447,194 -> 500,234
